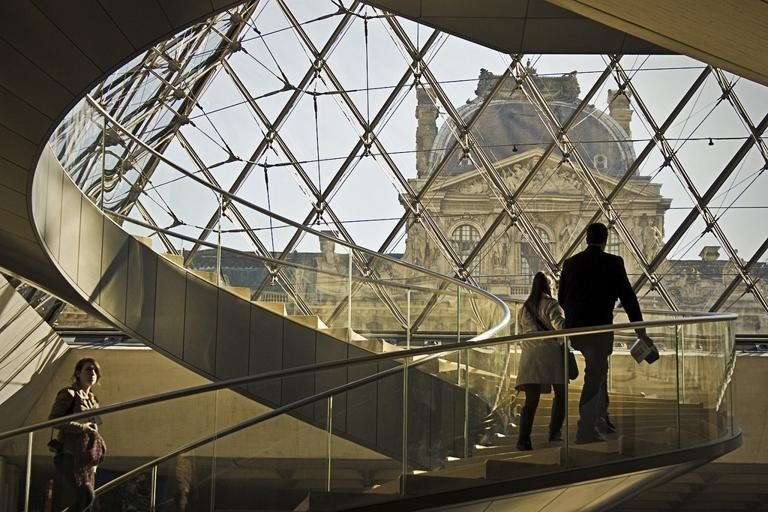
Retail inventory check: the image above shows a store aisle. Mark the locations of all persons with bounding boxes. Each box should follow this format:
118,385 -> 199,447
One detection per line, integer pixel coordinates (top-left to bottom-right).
46,356 -> 107,510
559,221 -> 654,445
516,270 -> 571,451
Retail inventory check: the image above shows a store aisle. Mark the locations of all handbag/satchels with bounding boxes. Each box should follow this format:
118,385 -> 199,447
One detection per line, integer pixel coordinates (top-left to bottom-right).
561,344 -> 579,381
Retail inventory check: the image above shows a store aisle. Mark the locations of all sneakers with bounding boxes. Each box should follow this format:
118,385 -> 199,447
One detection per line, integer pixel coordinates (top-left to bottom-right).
517,414 -> 617,452
